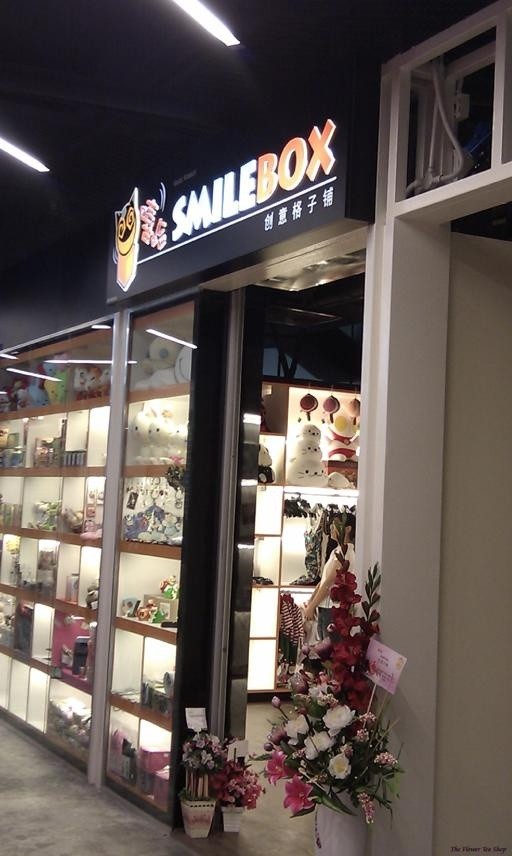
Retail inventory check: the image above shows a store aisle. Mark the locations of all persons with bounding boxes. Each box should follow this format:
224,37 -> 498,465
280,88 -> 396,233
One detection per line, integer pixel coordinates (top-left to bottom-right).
305,512 -> 355,640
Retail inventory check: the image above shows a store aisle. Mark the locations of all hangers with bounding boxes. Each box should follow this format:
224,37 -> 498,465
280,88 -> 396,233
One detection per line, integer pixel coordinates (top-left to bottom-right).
279,588 -> 292,599
282,489 -> 358,524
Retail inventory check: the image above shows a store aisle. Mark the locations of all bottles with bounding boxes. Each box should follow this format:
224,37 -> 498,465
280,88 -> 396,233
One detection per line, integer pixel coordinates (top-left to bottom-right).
3,434 -> 26,468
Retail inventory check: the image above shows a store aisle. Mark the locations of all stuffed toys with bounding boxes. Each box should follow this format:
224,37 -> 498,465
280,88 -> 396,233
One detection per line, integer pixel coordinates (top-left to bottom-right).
128,402 -> 187,465
285,402 -> 358,489
134,333 -> 192,389
0,351 -> 111,416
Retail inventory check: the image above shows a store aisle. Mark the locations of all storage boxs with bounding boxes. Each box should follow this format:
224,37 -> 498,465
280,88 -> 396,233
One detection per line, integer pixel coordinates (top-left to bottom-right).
322,459 -> 357,489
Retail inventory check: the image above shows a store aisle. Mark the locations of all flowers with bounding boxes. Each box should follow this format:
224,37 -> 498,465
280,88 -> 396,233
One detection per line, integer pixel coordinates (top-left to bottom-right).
254,551 -> 405,833
208,759 -> 267,813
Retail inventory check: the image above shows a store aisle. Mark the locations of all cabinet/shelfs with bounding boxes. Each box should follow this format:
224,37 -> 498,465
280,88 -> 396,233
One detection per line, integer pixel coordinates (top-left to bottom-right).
1,288 -> 229,832
245,381 -> 361,696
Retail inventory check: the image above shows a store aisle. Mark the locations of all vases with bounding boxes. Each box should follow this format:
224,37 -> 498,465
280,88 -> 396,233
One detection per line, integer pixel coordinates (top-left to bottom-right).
179,794 -> 216,840
311,781 -> 370,856
220,805 -> 245,833
